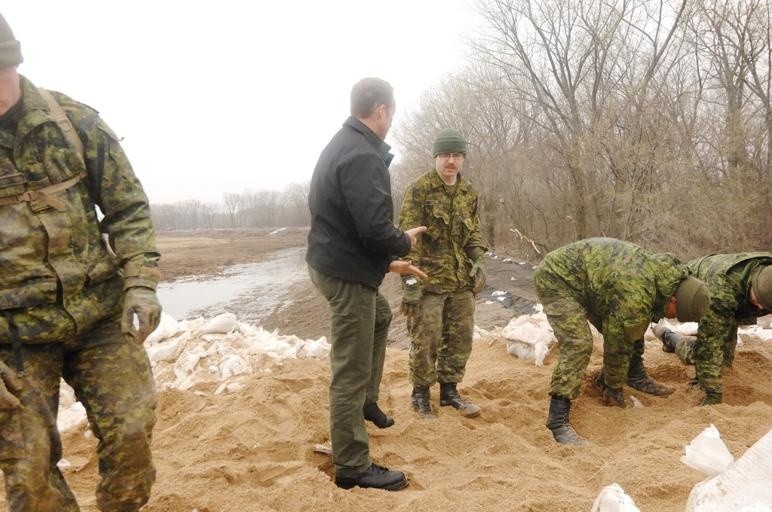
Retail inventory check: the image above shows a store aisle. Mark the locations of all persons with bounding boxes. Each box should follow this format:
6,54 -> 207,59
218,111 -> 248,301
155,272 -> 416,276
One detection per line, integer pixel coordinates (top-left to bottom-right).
397,128 -> 489,418
532,237 -> 711,445
653,251 -> 772,405
305,77 -> 428,491
0,14 -> 162,512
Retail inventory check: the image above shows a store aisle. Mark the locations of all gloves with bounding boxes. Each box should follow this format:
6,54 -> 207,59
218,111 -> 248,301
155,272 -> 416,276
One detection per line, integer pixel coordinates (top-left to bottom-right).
469,263 -> 487,292
122,262 -> 163,344
603,386 -> 626,408
400,276 -> 423,317
702,392 -> 721,405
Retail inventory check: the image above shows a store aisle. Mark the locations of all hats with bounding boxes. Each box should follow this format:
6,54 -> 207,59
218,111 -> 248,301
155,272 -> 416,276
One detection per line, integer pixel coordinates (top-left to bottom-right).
0,14 -> 24,69
752,264 -> 772,310
675,276 -> 710,322
433,128 -> 466,158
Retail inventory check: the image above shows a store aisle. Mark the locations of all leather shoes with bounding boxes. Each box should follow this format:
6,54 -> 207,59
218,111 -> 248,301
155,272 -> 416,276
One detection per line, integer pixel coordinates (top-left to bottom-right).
337,463 -> 408,491
363,402 -> 394,428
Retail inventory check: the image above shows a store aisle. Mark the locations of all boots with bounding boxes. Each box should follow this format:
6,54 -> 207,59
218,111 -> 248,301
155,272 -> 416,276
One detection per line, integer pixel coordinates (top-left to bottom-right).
412,385 -> 436,419
653,325 -> 682,352
440,382 -> 481,418
627,354 -> 675,395
546,395 -> 586,446
